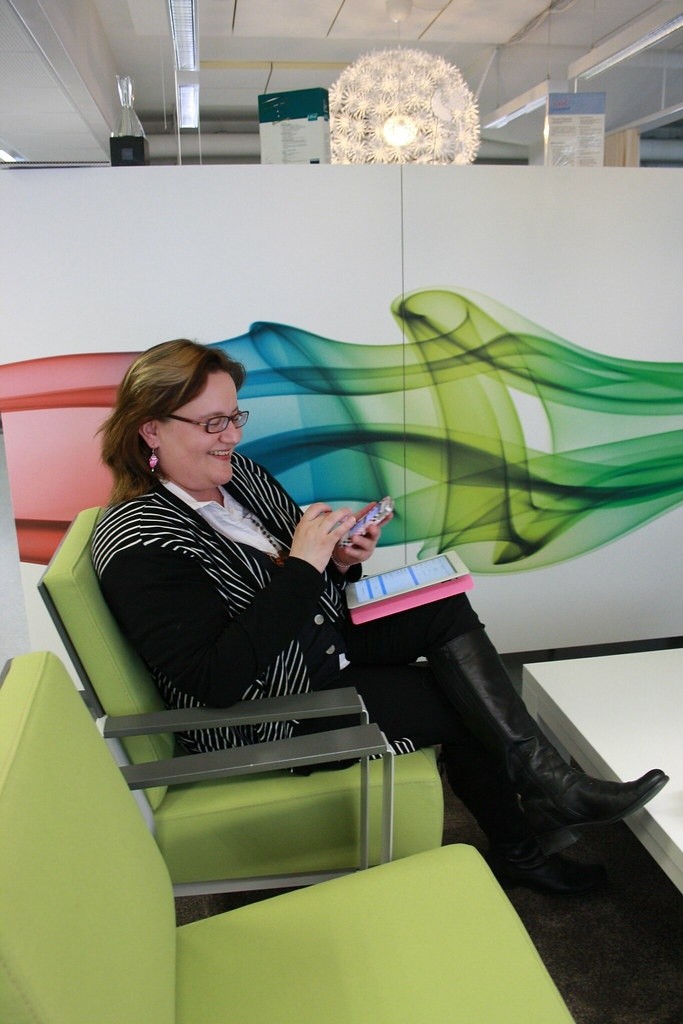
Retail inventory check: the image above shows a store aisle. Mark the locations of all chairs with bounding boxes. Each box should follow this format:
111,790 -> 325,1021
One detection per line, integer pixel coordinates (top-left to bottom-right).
0,648 -> 578,1024
40,505 -> 443,895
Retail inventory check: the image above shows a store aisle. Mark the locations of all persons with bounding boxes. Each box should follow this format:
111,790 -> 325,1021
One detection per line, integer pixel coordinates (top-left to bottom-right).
90,339 -> 670,897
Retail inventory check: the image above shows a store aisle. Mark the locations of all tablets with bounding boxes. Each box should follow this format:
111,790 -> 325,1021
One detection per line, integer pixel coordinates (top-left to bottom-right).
343,550 -> 471,610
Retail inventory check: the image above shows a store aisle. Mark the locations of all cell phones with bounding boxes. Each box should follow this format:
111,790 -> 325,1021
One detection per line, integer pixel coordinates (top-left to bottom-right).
337,496 -> 393,548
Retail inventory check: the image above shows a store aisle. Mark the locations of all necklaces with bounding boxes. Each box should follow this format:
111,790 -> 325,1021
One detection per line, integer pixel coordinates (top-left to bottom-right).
249,518 -> 289,568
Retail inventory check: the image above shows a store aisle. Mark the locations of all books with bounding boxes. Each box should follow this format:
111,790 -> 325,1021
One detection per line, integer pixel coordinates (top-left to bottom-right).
544,92 -> 605,167
258,88 -> 331,165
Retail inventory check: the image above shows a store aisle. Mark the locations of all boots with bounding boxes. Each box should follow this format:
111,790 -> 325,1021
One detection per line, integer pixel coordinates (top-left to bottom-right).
423,627 -> 669,858
436,740 -> 591,898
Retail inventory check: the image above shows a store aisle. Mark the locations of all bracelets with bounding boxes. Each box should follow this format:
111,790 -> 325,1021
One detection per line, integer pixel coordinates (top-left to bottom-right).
331,552 -> 350,568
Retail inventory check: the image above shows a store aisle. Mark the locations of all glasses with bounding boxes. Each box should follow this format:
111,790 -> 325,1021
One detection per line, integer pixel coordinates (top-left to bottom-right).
166,411 -> 249,434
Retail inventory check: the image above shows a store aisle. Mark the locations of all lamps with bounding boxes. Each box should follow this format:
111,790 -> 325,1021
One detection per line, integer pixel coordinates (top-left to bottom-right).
571,44 -> 683,134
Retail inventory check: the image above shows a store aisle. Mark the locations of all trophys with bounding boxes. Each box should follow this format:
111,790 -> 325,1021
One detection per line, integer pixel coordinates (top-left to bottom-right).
108,76 -> 149,166
